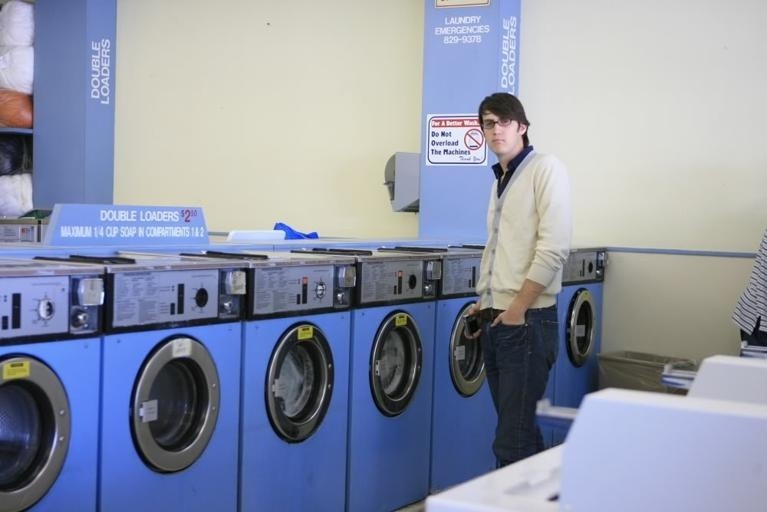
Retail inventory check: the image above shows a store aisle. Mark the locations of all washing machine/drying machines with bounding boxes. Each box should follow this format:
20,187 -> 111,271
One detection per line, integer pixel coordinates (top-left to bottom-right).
0,244 -> 612,512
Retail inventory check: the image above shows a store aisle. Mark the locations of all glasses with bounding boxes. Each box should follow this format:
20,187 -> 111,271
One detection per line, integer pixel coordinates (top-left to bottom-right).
481,117 -> 512,129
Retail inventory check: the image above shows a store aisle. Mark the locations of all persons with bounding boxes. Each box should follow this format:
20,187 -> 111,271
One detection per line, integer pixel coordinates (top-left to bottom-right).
731,225 -> 767,346
468,92 -> 572,471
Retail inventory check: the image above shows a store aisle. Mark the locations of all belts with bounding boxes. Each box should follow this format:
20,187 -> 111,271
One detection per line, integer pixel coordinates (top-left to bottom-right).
480,307 -> 506,320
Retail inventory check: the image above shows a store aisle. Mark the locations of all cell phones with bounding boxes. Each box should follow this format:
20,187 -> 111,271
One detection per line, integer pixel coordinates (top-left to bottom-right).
464,310 -> 481,335
